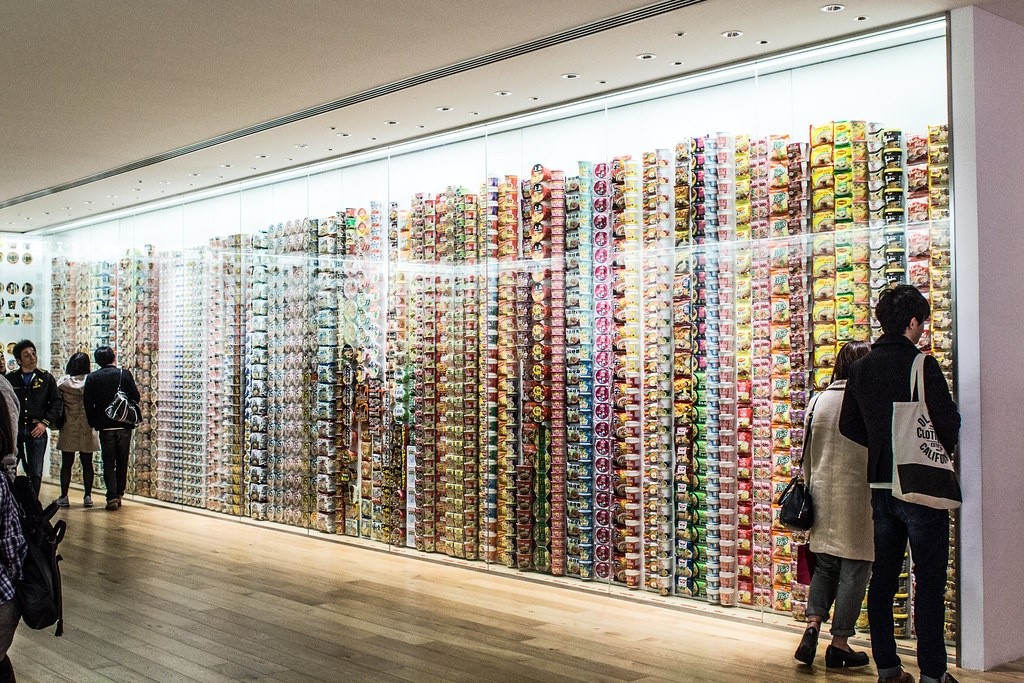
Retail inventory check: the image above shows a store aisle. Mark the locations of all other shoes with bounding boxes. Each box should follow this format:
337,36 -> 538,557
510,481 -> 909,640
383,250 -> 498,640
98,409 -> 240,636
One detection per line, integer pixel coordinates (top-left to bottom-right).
795,627 -> 818,665
919,672 -> 959,683
826,644 -> 869,669
878,665 -> 915,683
117,499 -> 121,507
105,499 -> 118,511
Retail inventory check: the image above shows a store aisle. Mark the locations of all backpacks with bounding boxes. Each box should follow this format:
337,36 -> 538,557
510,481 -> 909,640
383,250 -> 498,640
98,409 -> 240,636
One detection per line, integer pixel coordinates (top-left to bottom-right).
0,474 -> 62,629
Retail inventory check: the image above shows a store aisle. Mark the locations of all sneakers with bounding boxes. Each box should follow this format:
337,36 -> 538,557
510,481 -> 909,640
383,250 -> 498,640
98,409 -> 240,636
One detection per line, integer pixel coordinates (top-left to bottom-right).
52,496 -> 70,507
84,496 -> 93,507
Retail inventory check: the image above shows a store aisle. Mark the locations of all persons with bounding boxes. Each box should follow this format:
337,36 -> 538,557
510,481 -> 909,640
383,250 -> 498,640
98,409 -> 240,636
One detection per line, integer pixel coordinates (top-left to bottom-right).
838,285 -> 962,683
0,470 -> 29,683
52,352 -> 101,507
794,341 -> 875,668
0,339 -> 63,497
83,346 -> 140,510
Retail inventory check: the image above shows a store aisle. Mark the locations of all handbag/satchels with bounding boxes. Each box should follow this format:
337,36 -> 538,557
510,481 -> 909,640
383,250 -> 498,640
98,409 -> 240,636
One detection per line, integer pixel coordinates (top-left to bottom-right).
797,541 -> 817,585
105,369 -> 143,427
892,353 -> 962,510
778,476 -> 814,531
